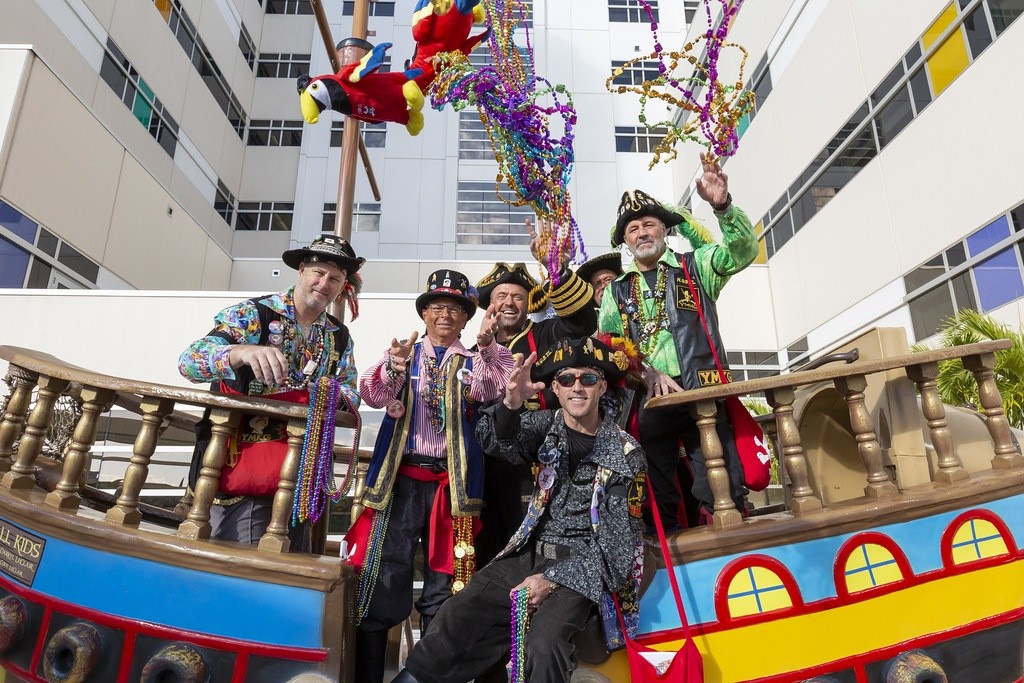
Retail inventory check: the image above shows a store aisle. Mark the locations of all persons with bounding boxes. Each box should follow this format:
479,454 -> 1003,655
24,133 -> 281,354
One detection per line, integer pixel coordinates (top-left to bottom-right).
173,156 -> 759,683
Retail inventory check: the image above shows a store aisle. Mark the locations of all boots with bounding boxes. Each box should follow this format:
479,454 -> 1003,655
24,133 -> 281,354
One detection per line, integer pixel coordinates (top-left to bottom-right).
355,631 -> 390,683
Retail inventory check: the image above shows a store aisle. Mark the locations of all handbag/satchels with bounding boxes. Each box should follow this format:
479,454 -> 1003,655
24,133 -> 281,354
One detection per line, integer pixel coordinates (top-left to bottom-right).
729,388 -> 773,492
622,634 -> 705,683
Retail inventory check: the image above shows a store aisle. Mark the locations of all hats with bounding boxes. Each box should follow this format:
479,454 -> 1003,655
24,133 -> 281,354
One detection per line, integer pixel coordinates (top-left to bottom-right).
575,252 -> 624,308
282,234 -> 368,321
611,189 -> 714,251
416,270 -> 478,324
531,326 -> 644,410
476,261 -> 548,314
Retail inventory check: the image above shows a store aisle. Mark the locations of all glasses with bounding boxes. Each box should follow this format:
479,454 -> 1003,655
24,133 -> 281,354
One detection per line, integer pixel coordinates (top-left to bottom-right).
426,303 -> 463,316
555,373 -> 604,386
